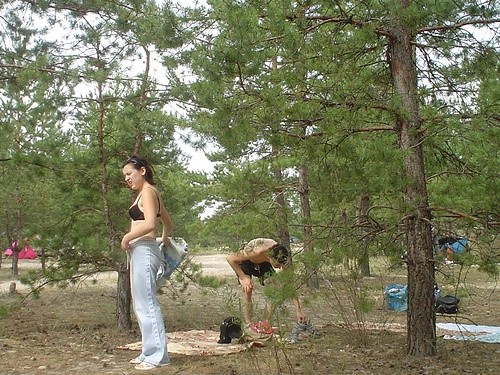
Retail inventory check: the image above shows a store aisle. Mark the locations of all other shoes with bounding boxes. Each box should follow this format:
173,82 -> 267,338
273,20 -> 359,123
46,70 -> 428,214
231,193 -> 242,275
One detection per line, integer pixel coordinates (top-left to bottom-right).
285,317 -> 325,345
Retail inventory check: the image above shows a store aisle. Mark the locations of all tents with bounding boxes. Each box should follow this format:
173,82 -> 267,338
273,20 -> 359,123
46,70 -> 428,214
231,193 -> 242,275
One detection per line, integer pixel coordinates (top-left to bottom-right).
5,239 -> 38,260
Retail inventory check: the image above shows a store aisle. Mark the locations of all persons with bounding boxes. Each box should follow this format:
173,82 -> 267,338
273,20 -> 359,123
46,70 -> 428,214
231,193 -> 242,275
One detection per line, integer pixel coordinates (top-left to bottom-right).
120,154 -> 174,371
226,238 -> 309,340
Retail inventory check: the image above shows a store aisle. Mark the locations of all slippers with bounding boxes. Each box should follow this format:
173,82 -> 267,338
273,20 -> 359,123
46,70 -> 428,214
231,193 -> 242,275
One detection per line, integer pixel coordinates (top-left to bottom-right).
128,357 -> 171,370
